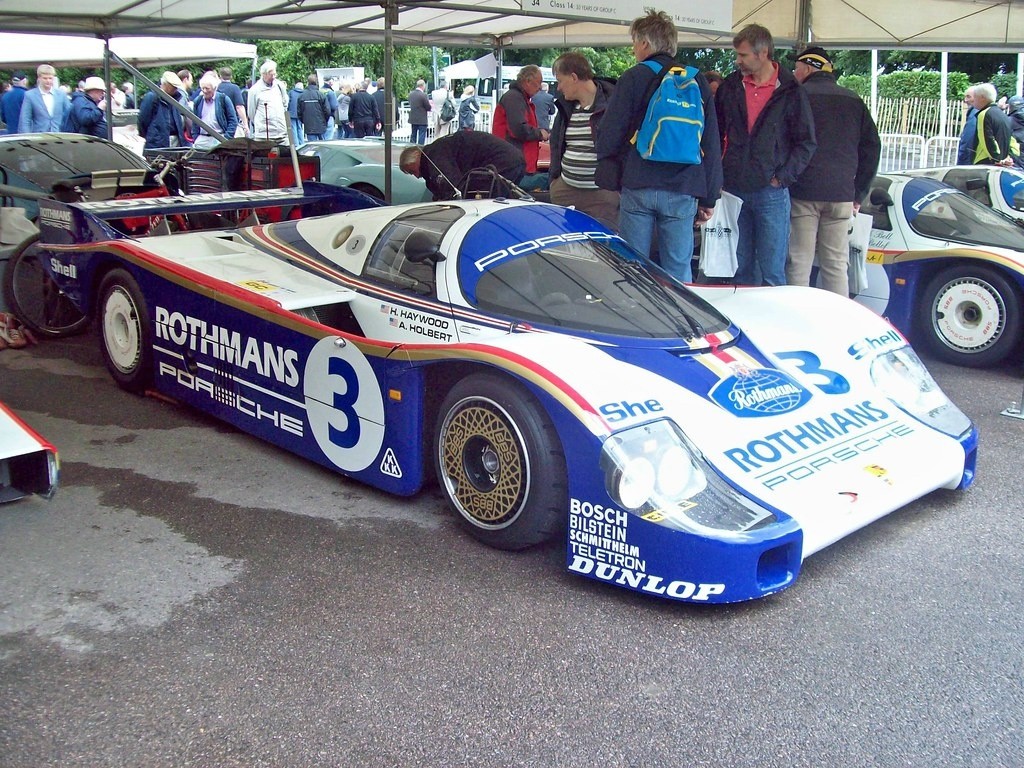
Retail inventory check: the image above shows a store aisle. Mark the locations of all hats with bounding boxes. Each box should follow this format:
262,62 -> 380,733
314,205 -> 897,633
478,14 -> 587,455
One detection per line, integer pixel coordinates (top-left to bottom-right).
465,85 -> 474,94
163,71 -> 183,88
84,76 -> 107,91
785,46 -> 834,72
323,74 -> 332,80
1005,95 -> 1024,105
17,73 -> 28,80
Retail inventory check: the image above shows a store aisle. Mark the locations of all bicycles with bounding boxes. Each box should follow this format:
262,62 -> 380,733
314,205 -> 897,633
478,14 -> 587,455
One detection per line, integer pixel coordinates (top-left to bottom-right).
2,149 -> 227,342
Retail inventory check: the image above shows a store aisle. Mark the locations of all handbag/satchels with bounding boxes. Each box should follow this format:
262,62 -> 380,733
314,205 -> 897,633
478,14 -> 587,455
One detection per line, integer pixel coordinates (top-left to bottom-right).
816,210 -> 873,294
440,90 -> 455,122
698,189 -> 743,277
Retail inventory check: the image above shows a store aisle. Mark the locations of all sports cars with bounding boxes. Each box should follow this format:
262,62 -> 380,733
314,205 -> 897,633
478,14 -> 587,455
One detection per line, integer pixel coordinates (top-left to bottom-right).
0,133 -> 168,285
294,139 -> 433,207
34,184 -> 977,605
807,164 -> 1024,365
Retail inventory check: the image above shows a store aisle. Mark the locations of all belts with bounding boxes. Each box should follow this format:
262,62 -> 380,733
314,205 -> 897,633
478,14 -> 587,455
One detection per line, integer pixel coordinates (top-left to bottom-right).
170,131 -> 178,135
201,133 -> 222,136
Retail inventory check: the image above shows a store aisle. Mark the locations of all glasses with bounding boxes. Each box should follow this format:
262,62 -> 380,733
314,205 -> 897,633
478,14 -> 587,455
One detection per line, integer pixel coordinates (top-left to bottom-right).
266,73 -> 278,77
13,79 -> 18,81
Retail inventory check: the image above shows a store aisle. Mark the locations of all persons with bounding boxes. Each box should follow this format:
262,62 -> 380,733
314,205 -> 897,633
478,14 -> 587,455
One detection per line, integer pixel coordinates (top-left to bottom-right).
400,130 -> 526,201
956,82 -> 1024,169
493,9 -> 882,299
407,78 -> 480,146
137,60 -> 400,157
1,64 -> 137,142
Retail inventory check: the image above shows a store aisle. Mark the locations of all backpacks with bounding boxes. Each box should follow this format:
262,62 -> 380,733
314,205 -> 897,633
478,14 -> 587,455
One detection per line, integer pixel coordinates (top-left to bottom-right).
636,60 -> 705,165
60,96 -> 90,133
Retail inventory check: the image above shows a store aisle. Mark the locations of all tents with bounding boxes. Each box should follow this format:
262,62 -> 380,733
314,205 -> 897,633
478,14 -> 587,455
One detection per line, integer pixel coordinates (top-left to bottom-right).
0,32 -> 258,110
